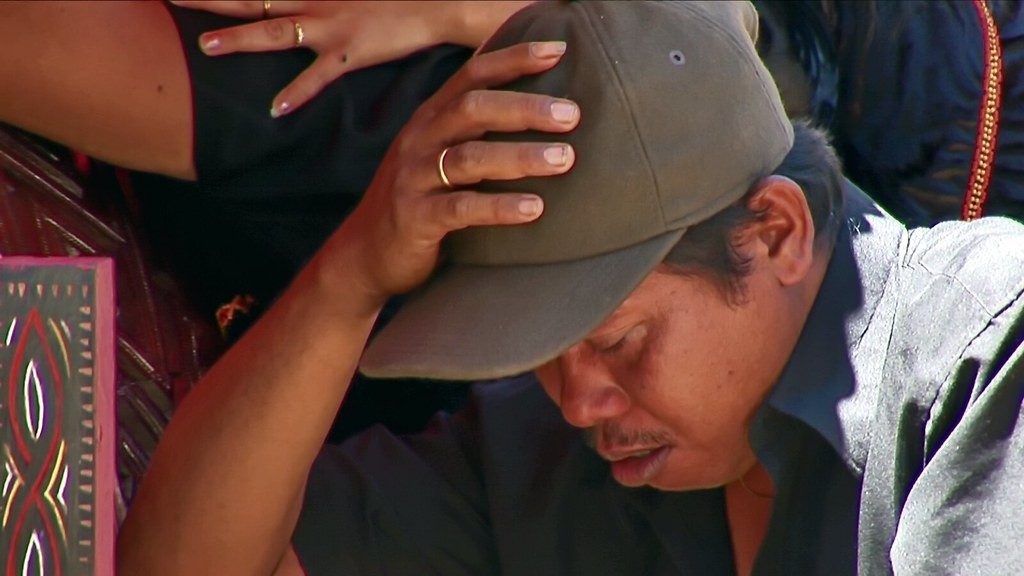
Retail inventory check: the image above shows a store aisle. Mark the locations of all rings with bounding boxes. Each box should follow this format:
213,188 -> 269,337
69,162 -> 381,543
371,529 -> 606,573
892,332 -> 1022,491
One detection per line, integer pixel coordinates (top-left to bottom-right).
289,16 -> 303,48
436,147 -> 462,186
262,0 -> 272,17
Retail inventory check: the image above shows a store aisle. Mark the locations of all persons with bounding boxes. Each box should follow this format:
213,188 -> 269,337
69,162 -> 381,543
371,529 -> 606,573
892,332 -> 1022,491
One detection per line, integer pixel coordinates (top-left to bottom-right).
115,0 -> 1024,576
0,0 -> 604,440
765,0 -> 1024,228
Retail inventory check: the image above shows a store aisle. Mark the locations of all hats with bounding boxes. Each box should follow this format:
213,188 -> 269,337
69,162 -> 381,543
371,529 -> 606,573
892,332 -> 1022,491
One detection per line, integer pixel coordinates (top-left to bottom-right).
360,0 -> 795,379
